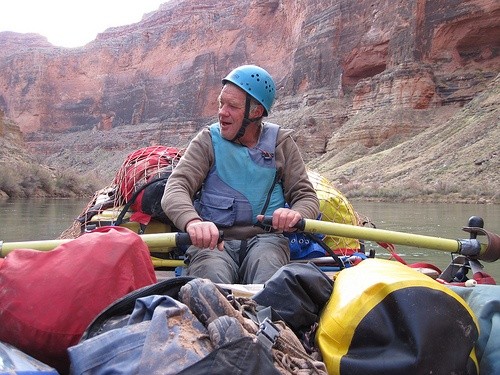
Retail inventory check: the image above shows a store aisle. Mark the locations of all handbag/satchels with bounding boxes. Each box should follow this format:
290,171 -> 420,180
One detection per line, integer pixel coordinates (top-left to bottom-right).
306,168 -> 365,259
313,257 -> 480,375
114,146 -> 184,225
0,223 -> 160,373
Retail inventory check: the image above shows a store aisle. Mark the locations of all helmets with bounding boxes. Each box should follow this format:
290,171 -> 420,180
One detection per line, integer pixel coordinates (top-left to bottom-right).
221,65 -> 276,117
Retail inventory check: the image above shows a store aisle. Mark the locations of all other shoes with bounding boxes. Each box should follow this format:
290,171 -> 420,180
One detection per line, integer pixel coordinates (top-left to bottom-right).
178,277 -> 310,360
206,314 -> 329,375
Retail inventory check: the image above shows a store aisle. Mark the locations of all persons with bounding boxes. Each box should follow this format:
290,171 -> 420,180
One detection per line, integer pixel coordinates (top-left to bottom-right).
160,63 -> 322,291
